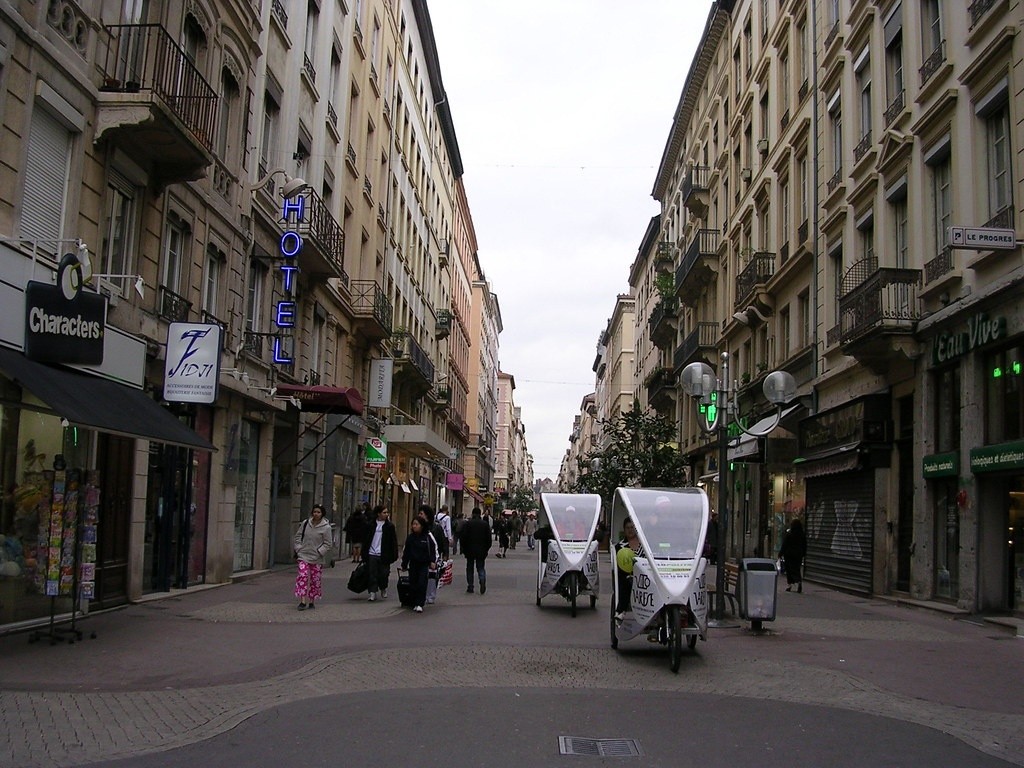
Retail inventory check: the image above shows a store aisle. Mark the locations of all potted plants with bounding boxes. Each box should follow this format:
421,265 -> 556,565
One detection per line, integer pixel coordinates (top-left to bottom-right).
392,326 -> 408,357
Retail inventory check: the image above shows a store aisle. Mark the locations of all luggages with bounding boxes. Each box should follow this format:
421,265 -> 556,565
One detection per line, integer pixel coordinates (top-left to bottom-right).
397,568 -> 411,607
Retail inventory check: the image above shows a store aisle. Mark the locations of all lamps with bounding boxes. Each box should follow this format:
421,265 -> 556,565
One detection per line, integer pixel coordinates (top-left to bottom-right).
435,369 -> 448,382
220,367 -> 301,409
733,305 -> 773,323
1,236 -> 92,285
477,445 -> 491,452
251,166 -> 308,199
92,273 -> 146,300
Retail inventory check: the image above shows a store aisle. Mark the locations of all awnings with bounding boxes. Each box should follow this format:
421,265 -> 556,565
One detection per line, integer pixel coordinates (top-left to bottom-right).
726,401 -> 802,461
277,382 -> 365,470
1,346 -> 220,453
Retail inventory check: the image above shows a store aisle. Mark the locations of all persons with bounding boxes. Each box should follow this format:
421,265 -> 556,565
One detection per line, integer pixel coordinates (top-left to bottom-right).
706,513 -> 720,566
359,505 -> 399,602
293,504 -> 333,610
450,508 -> 492,594
614,517 -> 646,620
418,505 -> 450,604
436,500 -> 527,558
642,495 -> 693,557
777,518 -> 807,593
524,513 -> 538,551
556,505 -> 587,541
401,516 -> 440,613
343,502 -> 374,562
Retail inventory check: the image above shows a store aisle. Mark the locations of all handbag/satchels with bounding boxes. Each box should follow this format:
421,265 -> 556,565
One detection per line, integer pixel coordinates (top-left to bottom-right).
347,560 -> 369,594
293,550 -> 299,560
776,558 -> 781,570
437,559 -> 454,589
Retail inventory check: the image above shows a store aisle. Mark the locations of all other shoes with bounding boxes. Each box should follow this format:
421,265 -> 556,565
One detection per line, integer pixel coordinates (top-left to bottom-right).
785,586 -> 791,591
381,589 -> 387,598
503,554 -> 506,559
615,611 -> 626,621
368,592 -> 377,601
495,553 -> 501,558
796,582 -> 802,593
428,598 -> 434,604
466,585 -> 474,593
308,603 -> 315,609
417,606 -> 423,611
298,602 -> 307,611
480,585 -> 486,594
413,606 -> 417,610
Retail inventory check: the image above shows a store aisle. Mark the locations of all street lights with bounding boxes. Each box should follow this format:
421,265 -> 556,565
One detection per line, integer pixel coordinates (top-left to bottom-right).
680,352 -> 799,629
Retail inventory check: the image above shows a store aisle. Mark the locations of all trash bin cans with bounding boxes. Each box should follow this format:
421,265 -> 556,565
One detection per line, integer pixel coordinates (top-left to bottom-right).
738,558 -> 778,621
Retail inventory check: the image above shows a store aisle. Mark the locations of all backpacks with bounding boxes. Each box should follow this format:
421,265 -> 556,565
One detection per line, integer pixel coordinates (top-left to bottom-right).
435,513 -> 446,530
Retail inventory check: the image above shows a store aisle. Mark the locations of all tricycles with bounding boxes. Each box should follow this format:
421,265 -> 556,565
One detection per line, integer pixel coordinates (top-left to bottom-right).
536,492 -> 602,617
609,486 -> 711,673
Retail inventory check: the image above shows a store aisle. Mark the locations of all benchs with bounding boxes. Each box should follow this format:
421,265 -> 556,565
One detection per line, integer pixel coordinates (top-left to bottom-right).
706,562 -> 738,616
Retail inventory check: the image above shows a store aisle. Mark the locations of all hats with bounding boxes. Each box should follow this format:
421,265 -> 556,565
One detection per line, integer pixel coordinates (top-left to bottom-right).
566,506 -> 576,512
655,496 -> 670,508
